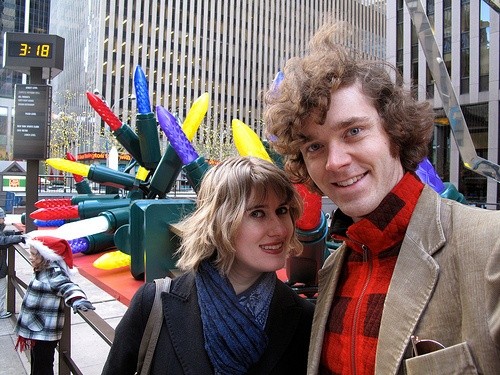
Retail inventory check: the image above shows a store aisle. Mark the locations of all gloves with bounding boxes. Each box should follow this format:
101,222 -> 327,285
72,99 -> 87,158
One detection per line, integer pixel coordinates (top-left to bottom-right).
72,298 -> 96,314
14,230 -> 25,235
21,236 -> 26,244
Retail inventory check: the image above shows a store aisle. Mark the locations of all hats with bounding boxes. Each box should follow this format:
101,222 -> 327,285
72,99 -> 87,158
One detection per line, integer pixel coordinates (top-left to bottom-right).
28,235 -> 79,278
0,207 -> 6,218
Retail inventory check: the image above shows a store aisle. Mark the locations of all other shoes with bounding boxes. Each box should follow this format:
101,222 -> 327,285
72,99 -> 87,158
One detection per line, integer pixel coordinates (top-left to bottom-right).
0,311 -> 12,319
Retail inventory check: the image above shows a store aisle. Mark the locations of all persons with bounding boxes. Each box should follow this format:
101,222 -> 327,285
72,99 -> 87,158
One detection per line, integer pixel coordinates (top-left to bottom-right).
101,156 -> 317,375
13,236 -> 96,375
0,207 -> 26,320
264,45 -> 500,375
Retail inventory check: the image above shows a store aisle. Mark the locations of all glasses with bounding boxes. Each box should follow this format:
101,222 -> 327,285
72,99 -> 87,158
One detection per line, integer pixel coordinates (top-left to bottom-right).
411,334 -> 446,358
